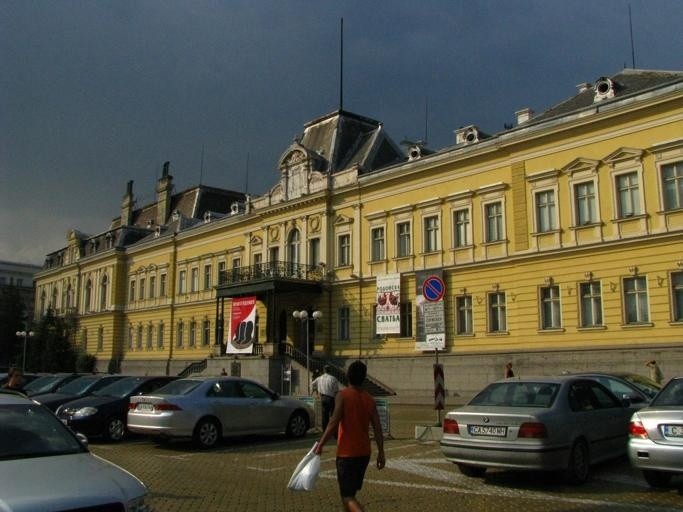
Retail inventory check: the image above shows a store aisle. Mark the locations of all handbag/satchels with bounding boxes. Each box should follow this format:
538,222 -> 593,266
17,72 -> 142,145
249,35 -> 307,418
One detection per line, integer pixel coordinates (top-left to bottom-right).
286,439 -> 321,493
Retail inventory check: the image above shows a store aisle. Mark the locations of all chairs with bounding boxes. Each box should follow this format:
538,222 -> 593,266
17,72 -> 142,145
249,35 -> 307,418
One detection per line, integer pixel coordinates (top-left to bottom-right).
517,391 -> 531,404
534,387 -> 553,405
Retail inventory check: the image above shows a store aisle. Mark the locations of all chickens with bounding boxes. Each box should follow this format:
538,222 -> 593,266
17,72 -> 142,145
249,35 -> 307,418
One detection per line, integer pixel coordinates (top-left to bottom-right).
377,292 -> 387,306
389,292 -> 399,306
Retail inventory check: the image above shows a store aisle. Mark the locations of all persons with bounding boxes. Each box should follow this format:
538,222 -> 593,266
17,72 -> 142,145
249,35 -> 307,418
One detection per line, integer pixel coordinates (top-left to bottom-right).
221,368 -> 227,376
645,359 -> 661,384
310,364 -> 339,440
314,360 -> 385,512
311,369 -> 320,396
1,367 -> 27,395
505,363 -> 514,378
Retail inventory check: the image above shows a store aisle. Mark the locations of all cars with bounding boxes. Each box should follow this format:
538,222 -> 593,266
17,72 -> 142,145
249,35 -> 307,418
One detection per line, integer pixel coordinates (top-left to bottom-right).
440,372 -> 683,489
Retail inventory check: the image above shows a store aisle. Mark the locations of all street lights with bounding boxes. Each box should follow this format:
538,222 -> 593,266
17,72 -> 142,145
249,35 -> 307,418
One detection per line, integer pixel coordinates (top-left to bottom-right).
14,330 -> 34,373
291,309 -> 323,396
